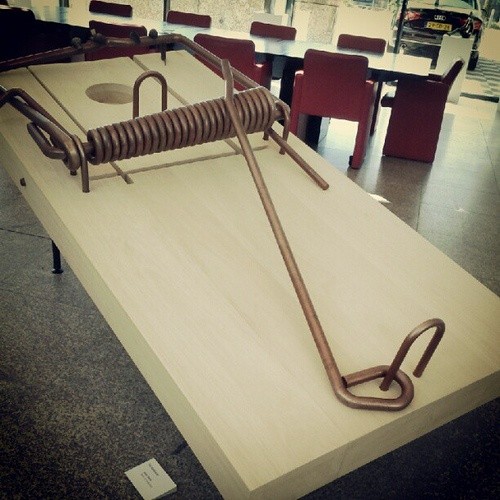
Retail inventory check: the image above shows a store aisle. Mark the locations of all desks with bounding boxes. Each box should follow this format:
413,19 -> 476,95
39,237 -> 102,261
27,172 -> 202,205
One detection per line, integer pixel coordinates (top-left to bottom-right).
8,5 -> 433,136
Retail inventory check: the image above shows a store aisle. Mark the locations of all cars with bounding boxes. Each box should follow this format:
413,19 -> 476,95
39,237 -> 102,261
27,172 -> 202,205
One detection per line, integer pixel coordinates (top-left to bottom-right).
386,0 -> 483,71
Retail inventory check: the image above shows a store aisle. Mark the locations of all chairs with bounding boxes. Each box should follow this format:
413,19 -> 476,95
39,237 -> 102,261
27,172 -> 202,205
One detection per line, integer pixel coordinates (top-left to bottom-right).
165,10 -> 211,29
84,18 -> 158,62
290,47 -> 380,169
192,32 -> 272,95
251,20 -> 296,42
380,57 -> 465,163
89,0 -> 132,18
337,33 -> 387,55
